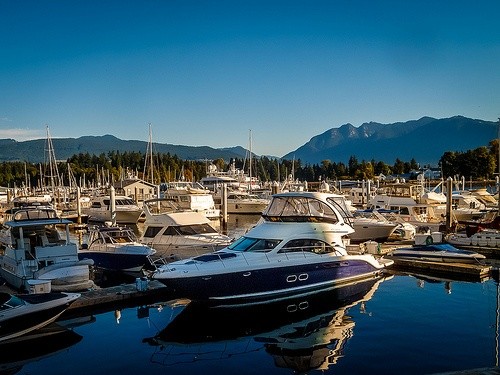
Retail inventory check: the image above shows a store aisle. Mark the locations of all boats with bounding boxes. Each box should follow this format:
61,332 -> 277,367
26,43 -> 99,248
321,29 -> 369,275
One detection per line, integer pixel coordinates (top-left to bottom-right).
141,192 -> 395,307
132,199 -> 244,260
0,120 -> 500,246
0,208 -> 94,292
387,241 -> 486,265
0,292 -> 81,342
77,228 -> 157,274
